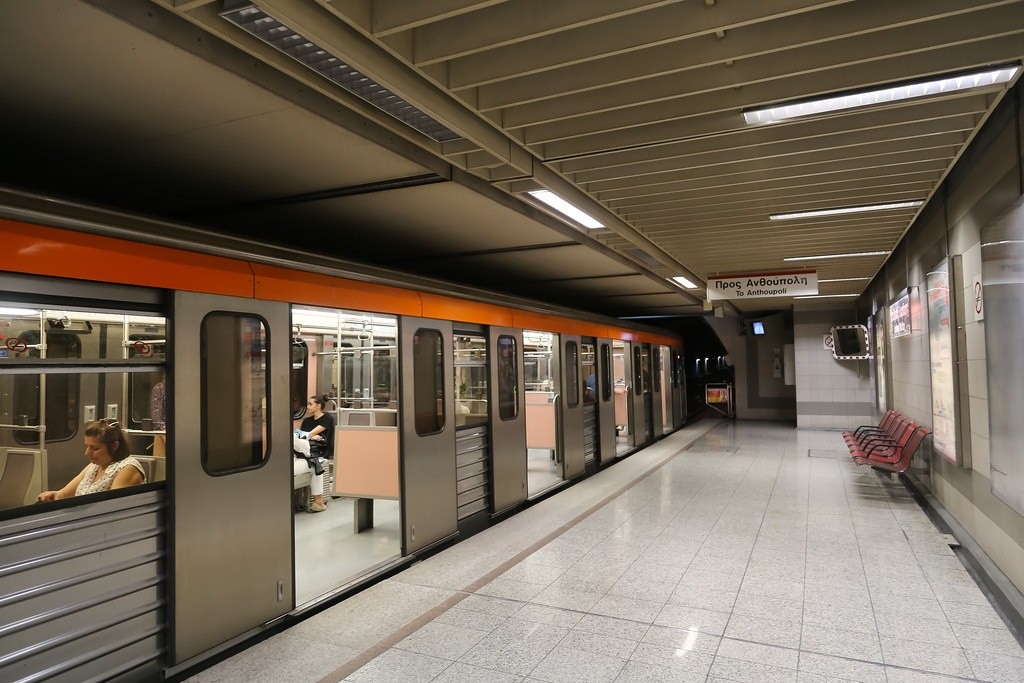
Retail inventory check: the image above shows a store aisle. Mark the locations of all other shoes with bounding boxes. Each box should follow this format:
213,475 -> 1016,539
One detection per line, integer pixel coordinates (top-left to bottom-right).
310,503 -> 327,511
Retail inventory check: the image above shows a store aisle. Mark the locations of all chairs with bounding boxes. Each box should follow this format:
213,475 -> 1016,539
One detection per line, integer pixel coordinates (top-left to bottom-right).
294,399 -> 488,534
0,449 -> 165,510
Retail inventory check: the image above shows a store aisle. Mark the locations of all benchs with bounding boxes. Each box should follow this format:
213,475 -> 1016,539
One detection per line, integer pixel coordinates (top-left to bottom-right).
331,425 -> 399,534
842,409 -> 934,482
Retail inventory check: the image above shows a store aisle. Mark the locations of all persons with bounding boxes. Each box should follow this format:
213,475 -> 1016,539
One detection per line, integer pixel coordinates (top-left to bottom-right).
293,432 -> 327,512
149,370 -> 166,457
300,394 -> 334,457
36,416 -> 145,503
577,372 -> 597,405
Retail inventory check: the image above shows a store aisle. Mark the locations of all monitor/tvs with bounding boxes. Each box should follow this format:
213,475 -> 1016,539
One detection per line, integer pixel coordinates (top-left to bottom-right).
752,321 -> 765,335
